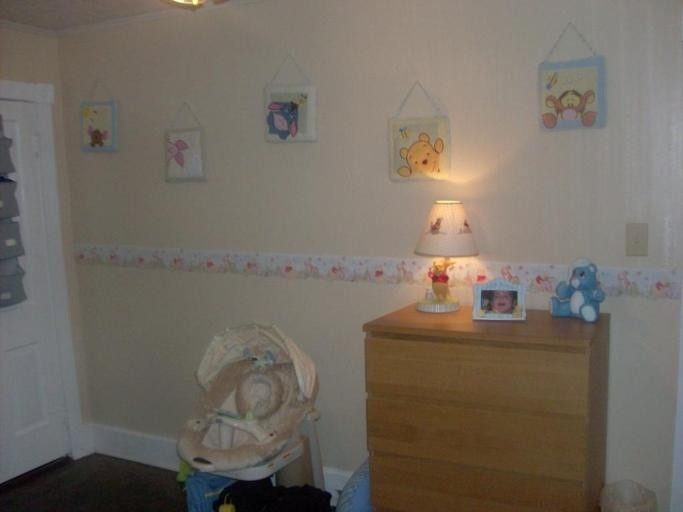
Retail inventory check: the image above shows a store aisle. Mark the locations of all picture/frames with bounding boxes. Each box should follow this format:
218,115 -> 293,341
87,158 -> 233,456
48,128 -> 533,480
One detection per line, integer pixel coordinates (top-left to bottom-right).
80,101 -> 116,152
538,58 -> 605,130
263,86 -> 317,142
164,127 -> 205,182
473,277 -> 526,320
388,117 -> 451,182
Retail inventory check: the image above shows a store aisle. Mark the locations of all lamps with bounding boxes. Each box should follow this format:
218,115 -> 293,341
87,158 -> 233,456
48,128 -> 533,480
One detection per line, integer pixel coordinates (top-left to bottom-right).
413,200 -> 480,313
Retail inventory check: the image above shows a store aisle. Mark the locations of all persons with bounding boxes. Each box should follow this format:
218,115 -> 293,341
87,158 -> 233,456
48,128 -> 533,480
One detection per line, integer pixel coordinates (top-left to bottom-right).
488,290 -> 514,312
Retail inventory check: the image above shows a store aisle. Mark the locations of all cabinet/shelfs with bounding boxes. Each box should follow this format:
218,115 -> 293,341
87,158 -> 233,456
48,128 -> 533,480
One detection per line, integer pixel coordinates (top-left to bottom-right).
362,301 -> 610,512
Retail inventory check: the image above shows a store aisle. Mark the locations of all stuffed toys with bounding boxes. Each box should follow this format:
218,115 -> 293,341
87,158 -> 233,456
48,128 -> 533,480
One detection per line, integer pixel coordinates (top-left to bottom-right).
549,263 -> 604,322
427,261 -> 454,305
